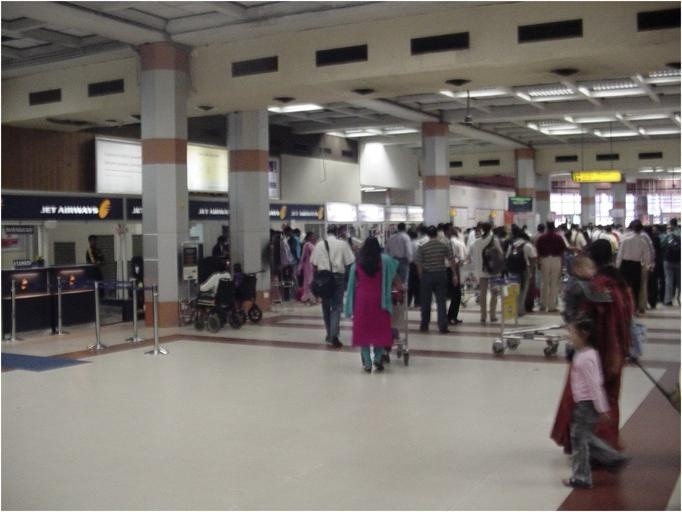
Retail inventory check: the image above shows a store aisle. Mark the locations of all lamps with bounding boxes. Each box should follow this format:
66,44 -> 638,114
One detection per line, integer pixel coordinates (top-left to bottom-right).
572,122 -> 623,182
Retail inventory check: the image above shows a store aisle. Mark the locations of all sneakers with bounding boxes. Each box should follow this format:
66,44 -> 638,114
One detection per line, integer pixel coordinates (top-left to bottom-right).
562,475 -> 594,489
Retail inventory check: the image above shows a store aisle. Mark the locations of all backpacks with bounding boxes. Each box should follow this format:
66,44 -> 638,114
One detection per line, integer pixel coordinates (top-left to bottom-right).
505,241 -> 530,281
482,238 -> 505,275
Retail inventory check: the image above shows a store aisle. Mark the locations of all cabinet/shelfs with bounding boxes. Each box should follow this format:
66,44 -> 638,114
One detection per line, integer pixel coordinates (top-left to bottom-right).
1,264 -> 96,339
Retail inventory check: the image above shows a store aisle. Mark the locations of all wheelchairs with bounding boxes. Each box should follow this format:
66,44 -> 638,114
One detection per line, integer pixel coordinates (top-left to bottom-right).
232,269 -> 266,322
191,275 -> 242,333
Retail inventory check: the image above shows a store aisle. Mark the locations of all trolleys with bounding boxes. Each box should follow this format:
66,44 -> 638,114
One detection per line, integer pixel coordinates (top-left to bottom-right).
383,288 -> 409,366
492,283 -> 572,357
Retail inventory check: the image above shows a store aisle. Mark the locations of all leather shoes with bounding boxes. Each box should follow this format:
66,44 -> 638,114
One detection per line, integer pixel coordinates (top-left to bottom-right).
374,360 -> 385,372
364,367 -> 373,373
326,335 -> 332,344
332,338 -> 343,348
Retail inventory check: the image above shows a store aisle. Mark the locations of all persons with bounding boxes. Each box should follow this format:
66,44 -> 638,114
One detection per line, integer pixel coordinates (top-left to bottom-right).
297,231 -> 318,306
658,219 -> 681,306
495,223 -> 532,241
505,229 -> 537,318
344,235 -> 407,374
537,217 -> 581,313
385,223 -> 412,309
211,236 -> 230,274
467,222 -> 485,246
640,231 -> 655,309
415,226 -> 458,335
561,256 -> 613,362
470,223 -> 503,324
438,223 -> 463,324
276,228 -> 301,300
232,263 -> 244,295
201,259 -> 231,298
561,318 -> 630,489
308,223 -> 355,347
561,216 -> 626,248
616,221 -> 649,313
551,239 -> 634,464
85,235 -> 103,262
533,223 -> 545,245
